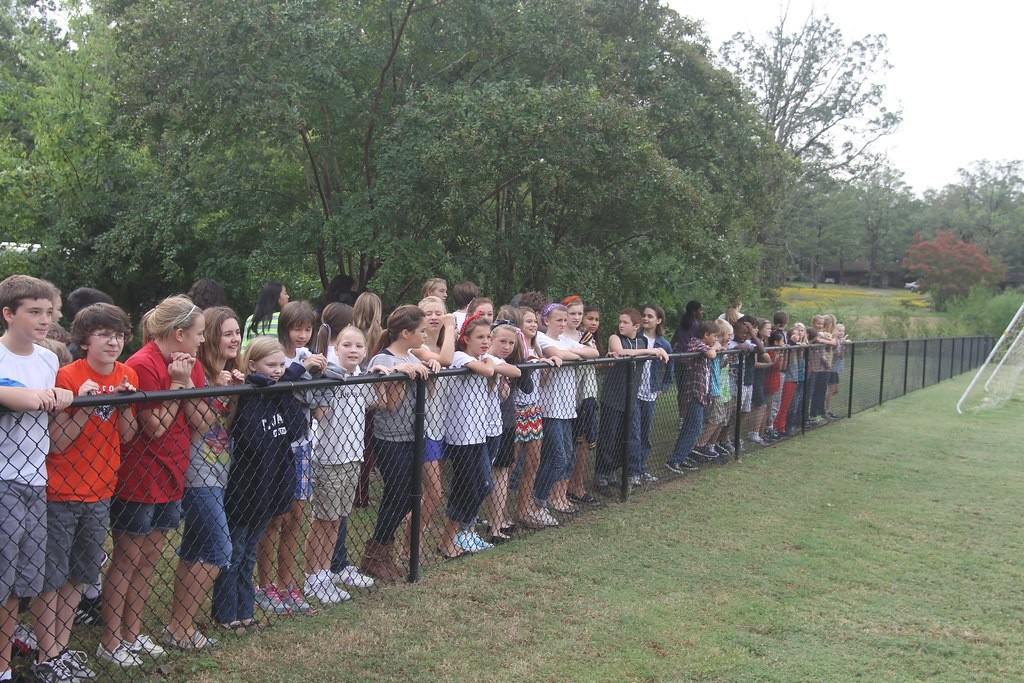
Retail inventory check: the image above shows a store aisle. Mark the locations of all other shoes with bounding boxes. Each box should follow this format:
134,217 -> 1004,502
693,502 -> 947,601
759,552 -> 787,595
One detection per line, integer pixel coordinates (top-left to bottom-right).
214,618 -> 260,629
547,501 -> 580,513
437,544 -> 470,558
368,500 -> 377,505
487,524 -> 517,543
101,553 -> 108,565
474,515 -> 488,523
532,508 -> 558,526
594,481 -> 622,497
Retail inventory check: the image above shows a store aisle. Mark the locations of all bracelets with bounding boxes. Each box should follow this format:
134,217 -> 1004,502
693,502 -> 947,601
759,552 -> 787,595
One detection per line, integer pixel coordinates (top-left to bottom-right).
172,382 -> 184,385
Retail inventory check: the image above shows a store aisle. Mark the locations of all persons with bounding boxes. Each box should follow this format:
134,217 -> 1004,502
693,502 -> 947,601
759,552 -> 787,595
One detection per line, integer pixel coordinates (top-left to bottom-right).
666,301 -> 852,473
163,306 -> 245,649
360,277 -> 674,582
188,279 -> 224,311
211,335 -> 306,637
31,302 -> 138,683
353,292 -> 382,508
96,297 -> 215,665
316,303 -> 373,587
0,275 -> 73,683
253,301 -> 347,615
316,275 -> 360,315
304,326 -> 391,604
11,288 -> 114,658
240,283 -> 289,354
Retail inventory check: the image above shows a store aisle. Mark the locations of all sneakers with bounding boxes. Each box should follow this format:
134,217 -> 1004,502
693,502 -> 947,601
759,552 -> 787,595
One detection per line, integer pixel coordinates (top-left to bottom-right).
663,411 -> 840,473
254,582 -> 313,615
73,599 -> 107,626
12,623 -> 39,649
567,490 -> 600,506
163,626 -> 219,649
452,531 -> 495,552
329,566 -> 374,587
304,579 -> 350,604
32,648 -> 97,683
630,473 -> 658,486
96,635 -> 166,666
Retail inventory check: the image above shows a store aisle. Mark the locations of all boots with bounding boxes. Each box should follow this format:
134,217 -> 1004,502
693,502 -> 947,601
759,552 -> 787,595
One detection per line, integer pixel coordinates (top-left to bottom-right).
360,536 -> 406,581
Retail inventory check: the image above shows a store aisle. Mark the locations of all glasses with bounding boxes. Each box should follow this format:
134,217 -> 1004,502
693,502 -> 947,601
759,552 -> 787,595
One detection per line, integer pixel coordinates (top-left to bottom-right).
91,331 -> 130,344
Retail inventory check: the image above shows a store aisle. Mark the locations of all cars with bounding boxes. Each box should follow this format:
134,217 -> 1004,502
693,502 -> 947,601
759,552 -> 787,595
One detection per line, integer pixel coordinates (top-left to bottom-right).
905,281 -> 921,292
